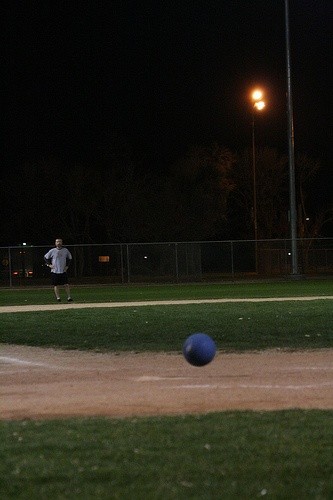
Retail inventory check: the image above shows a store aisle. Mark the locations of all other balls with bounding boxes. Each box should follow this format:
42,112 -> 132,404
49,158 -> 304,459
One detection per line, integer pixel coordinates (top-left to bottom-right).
184,333 -> 217,367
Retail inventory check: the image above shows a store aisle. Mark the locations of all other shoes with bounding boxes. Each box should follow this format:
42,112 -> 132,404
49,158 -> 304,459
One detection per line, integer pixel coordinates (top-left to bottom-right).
67,299 -> 73,302
57,298 -> 62,303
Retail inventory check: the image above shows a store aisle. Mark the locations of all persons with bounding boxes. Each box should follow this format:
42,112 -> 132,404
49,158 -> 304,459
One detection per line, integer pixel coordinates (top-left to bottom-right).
43,238 -> 74,302
